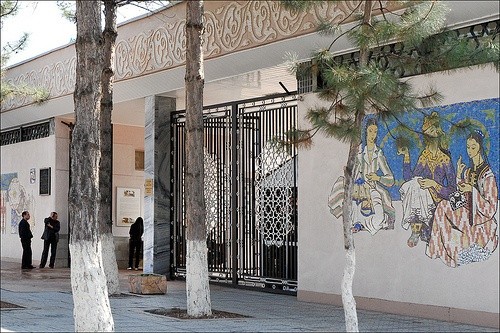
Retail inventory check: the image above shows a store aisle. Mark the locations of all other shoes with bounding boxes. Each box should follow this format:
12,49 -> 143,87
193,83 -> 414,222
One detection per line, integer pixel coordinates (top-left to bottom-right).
126,267 -> 132,270
135,268 -> 138,270
27,266 -> 36,269
49,263 -> 53,269
39,266 -> 44,268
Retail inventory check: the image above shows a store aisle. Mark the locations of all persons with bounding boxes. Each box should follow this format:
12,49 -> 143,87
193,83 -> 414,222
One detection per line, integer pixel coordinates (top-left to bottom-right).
126,217 -> 144,271
39,212 -> 60,269
18,211 -> 36,269
